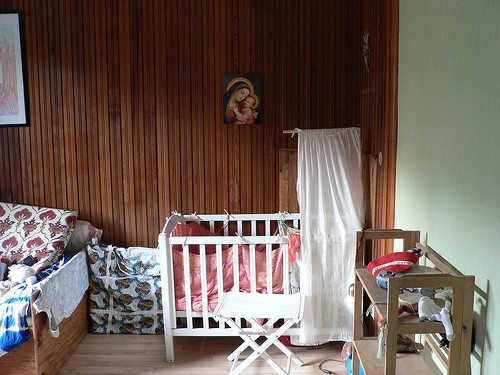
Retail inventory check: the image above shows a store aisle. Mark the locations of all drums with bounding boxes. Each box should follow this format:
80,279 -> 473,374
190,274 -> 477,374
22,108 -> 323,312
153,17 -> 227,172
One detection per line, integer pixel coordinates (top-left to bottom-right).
377,293 -> 423,327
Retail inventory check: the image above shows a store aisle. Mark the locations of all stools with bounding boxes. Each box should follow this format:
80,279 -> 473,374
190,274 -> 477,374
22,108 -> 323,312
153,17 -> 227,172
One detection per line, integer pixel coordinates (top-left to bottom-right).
211,292 -> 306,375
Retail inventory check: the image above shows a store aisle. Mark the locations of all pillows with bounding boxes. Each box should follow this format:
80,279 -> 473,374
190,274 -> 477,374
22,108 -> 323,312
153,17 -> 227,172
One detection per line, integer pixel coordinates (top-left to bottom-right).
0,202 -> 77,268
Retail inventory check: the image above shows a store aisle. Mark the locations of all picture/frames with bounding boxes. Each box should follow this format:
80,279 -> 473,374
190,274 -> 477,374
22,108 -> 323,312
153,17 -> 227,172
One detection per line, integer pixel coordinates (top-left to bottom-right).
0,9 -> 32,127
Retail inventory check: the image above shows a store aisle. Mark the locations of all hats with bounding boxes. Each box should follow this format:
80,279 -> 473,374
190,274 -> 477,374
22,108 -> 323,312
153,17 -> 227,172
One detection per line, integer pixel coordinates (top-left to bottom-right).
367,251 -> 419,276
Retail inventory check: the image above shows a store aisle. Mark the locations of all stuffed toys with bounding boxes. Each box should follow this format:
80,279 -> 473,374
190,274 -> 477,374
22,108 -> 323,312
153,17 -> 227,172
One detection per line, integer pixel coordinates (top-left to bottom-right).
7,262 -> 37,289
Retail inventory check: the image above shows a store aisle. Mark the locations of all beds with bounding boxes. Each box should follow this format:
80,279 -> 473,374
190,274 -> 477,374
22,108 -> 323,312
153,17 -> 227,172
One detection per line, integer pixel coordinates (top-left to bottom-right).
158,210 -> 351,364
0,201 -> 102,375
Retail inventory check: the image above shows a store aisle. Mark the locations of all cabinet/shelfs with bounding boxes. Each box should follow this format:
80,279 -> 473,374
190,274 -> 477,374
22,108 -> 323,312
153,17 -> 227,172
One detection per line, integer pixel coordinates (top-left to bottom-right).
352,228 -> 476,375
